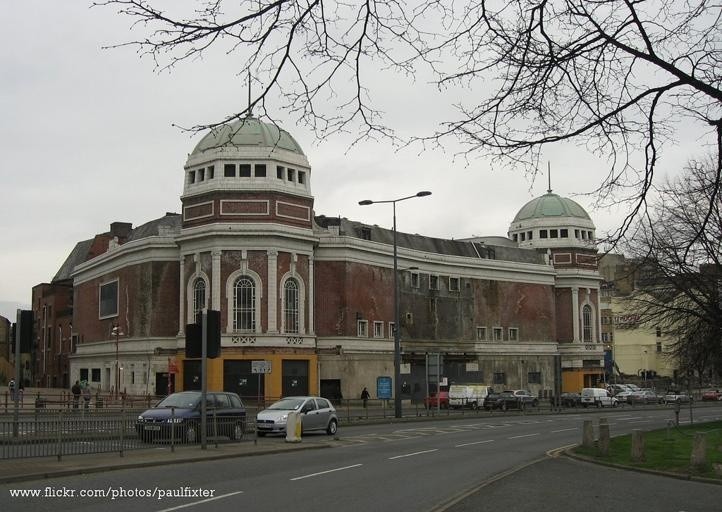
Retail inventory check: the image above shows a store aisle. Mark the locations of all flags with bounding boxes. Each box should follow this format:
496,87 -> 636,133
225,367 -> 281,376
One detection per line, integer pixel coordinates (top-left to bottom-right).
169,359 -> 180,374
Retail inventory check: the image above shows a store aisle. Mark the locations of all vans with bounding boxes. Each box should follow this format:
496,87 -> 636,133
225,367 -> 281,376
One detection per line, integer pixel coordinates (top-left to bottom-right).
134,390 -> 246,444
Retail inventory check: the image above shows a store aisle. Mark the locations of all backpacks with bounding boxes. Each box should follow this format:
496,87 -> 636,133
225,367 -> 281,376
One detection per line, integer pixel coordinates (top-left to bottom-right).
9,380 -> 15,387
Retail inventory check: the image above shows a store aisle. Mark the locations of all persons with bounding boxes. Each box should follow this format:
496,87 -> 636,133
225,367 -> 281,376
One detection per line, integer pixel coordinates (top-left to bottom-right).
83,383 -> 92,411
8,377 -> 17,401
361,386 -> 370,408
72,379 -> 82,409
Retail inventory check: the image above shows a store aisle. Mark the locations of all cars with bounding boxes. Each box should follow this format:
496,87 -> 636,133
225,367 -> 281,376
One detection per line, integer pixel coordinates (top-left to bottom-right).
424,384 -> 540,412
254,396 -> 341,438
702,389 -> 722,401
550,383 -> 693,408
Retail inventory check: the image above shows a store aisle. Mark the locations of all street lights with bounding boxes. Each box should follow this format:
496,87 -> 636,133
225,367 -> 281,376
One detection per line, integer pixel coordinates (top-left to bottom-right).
111,326 -> 124,400
358,191 -> 432,416
